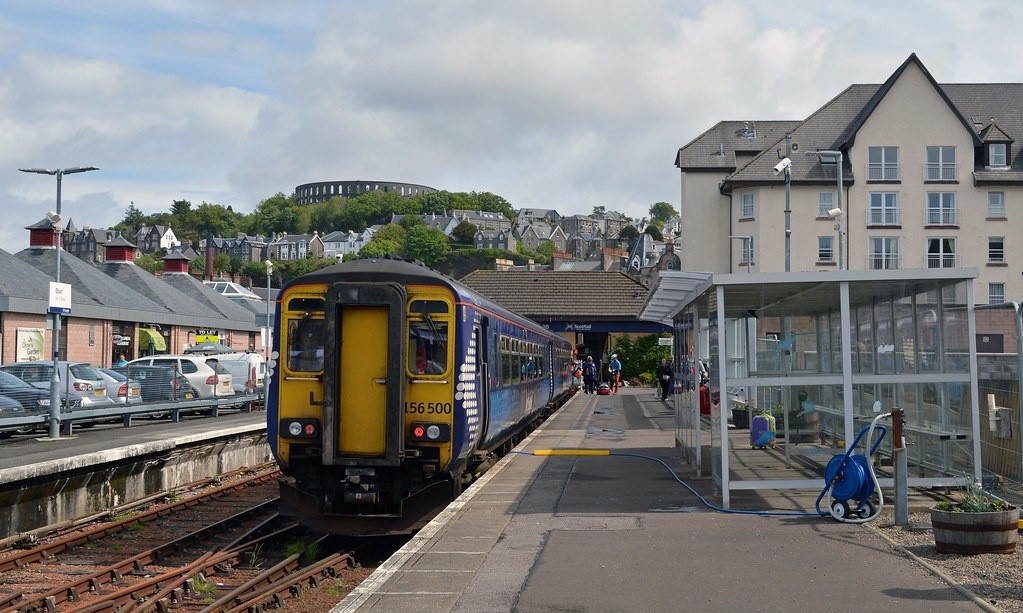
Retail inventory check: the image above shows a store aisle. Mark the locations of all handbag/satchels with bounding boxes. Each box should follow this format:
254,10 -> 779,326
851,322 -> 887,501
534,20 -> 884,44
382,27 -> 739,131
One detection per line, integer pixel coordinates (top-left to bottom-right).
582,362 -> 589,376
572,363 -> 583,379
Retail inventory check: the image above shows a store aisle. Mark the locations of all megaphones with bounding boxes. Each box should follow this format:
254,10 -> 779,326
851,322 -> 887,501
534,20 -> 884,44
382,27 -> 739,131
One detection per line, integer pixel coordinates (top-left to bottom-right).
791,142 -> 799,153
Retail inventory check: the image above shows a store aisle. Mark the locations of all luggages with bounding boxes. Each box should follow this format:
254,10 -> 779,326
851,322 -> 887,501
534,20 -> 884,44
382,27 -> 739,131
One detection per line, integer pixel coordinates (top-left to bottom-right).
698,377 -> 711,417
597,383 -> 610,395
749,386 -> 776,450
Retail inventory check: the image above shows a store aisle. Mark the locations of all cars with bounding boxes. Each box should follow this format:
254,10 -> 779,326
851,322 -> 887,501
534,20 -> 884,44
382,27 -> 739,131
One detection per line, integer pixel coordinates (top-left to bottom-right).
119,353 -> 235,416
0,360 -> 108,428
111,367 -> 196,419
0,353 -> 83,439
96,366 -> 143,423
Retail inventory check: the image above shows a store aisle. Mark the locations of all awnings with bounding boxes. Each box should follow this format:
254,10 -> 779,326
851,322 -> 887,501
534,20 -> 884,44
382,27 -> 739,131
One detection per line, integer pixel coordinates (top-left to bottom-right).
139,327 -> 167,352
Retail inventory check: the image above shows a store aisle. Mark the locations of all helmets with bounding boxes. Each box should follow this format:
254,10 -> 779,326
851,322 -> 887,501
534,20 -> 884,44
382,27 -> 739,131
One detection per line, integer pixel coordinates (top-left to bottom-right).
611,354 -> 617,360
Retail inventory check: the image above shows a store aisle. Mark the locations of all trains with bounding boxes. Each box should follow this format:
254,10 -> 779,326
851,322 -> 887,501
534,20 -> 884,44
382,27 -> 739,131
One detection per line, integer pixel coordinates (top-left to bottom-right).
267,253 -> 573,539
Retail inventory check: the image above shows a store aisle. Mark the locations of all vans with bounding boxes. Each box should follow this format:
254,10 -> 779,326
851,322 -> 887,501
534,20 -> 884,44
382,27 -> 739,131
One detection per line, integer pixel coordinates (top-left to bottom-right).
189,352 -> 266,410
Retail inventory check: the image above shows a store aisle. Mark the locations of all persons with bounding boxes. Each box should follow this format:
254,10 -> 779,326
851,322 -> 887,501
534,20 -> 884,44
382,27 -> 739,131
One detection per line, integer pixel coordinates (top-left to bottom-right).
563,361 -> 571,387
608,353 -> 621,395
681,355 -> 690,393
582,356 -> 598,394
795,395 -> 815,418
27,356 -> 40,378
526,356 -> 537,379
692,356 -> 705,390
658,356 -> 674,401
113,353 -> 128,368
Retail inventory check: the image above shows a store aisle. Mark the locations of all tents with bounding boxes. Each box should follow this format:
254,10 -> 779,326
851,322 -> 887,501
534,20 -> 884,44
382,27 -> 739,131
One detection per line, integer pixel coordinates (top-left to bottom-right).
184,341 -> 236,355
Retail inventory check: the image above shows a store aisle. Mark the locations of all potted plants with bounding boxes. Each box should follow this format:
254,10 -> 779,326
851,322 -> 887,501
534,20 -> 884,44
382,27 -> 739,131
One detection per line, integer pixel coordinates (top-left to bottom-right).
930,471 -> 1020,555
766,403 -> 792,430
732,403 -> 763,428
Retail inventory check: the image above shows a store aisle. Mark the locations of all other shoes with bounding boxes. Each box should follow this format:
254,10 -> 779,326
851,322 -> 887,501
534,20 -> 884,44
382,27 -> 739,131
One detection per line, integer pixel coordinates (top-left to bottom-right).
662,397 -> 665,401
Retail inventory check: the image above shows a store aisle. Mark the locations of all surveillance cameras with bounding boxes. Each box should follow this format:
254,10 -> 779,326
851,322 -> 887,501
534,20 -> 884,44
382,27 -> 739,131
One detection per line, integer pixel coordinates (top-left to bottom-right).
774,157 -> 791,175
336,254 -> 343,260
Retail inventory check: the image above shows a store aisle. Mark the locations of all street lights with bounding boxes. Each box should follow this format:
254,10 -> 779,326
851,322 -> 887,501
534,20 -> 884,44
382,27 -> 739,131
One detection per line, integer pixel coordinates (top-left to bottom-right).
805,151 -> 844,269
18,167 -> 101,437
